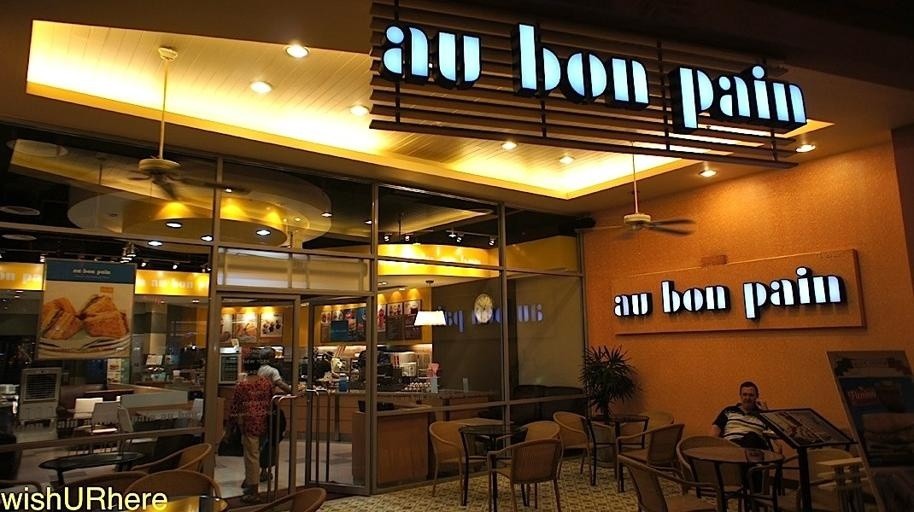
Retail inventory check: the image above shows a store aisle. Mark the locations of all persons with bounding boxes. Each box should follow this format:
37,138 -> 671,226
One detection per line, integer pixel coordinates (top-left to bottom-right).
227,352 -> 274,503
13,346 -> 26,364
241,347 -> 305,488
349,369 -> 359,383
709,381 -> 799,512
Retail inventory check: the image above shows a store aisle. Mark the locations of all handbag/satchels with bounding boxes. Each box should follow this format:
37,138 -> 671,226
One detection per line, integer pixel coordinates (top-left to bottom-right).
266,410 -> 286,439
218,431 -> 242,457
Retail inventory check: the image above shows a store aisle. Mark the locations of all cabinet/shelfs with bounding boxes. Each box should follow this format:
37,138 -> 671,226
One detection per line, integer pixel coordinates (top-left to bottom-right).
352,402 -> 433,488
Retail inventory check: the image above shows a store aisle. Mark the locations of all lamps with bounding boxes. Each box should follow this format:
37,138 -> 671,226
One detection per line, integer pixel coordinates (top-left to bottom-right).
414,279 -> 448,327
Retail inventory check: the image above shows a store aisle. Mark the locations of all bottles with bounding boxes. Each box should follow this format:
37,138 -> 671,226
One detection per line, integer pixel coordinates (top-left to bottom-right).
339,374 -> 347,392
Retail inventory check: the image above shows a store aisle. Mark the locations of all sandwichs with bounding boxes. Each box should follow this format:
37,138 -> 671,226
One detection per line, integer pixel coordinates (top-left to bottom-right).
40,293 -> 127,340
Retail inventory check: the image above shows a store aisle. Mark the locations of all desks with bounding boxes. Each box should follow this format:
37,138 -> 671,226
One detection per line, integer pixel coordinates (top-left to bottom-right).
84,388 -> 134,401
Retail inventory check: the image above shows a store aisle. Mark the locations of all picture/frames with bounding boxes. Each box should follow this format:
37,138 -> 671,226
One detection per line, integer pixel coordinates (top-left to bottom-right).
320,299 -> 422,342
220,312 -> 283,338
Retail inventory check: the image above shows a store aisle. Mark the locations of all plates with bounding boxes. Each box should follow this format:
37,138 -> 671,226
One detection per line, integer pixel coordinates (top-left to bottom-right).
38,331 -> 130,357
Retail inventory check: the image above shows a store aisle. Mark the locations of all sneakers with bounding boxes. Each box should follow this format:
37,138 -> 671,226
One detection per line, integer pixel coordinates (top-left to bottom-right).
260,473 -> 272,482
241,495 -> 260,503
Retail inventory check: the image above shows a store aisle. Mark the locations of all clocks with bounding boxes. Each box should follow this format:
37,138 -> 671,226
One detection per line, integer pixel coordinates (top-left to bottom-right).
473,293 -> 495,324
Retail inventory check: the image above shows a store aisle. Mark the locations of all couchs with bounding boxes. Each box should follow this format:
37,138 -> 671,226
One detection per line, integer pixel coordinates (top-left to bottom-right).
58,383 -> 105,427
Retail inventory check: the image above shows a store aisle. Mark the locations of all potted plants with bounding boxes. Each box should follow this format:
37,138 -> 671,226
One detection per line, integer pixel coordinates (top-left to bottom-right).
574,344 -> 643,467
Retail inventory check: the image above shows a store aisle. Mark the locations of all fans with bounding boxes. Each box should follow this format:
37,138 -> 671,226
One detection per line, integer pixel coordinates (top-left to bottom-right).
84,49 -> 251,202
573,141 -> 696,243
